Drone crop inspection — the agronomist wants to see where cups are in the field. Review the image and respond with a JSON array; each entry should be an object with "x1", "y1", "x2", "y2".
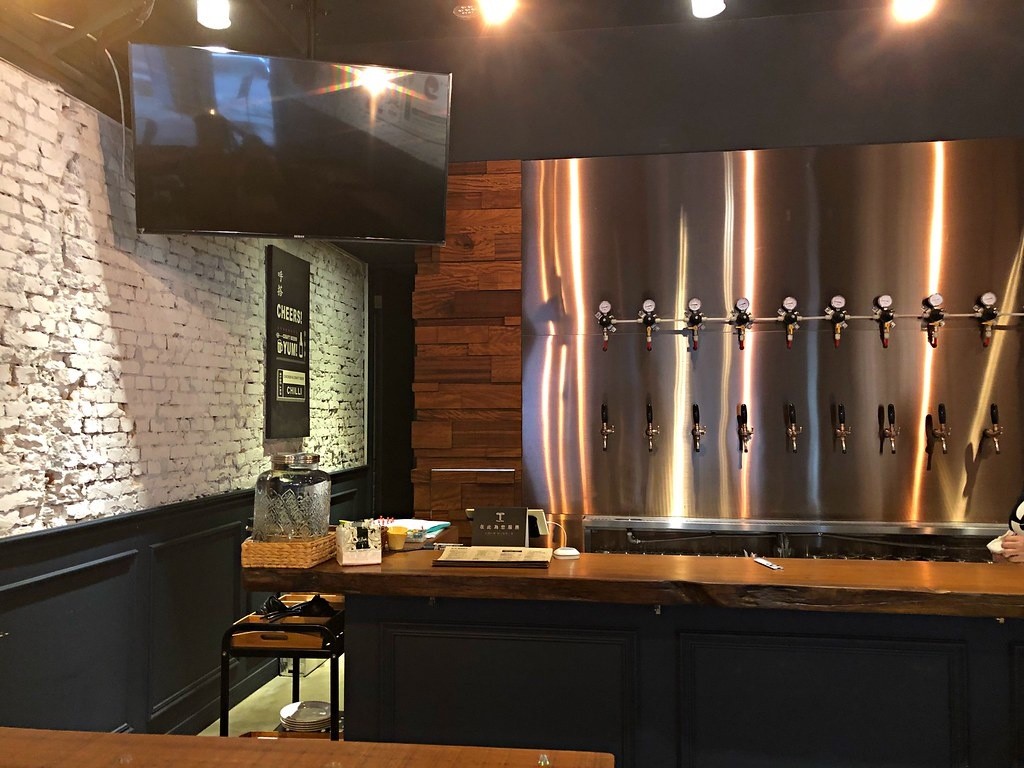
[
  {"x1": 381, "y1": 526, "x2": 389, "y2": 550},
  {"x1": 387, "y1": 526, "x2": 408, "y2": 550}
]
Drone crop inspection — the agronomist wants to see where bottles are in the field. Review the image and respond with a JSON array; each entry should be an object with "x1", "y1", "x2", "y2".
[{"x1": 253, "y1": 451, "x2": 331, "y2": 543}]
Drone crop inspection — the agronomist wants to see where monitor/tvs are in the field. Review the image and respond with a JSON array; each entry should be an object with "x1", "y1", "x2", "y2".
[{"x1": 128, "y1": 41, "x2": 452, "y2": 248}]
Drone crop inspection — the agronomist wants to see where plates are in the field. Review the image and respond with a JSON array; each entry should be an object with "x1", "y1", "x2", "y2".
[{"x1": 279, "y1": 700, "x2": 331, "y2": 732}]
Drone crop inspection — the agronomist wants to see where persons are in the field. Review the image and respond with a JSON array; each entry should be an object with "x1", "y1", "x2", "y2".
[{"x1": 991, "y1": 495, "x2": 1024, "y2": 563}]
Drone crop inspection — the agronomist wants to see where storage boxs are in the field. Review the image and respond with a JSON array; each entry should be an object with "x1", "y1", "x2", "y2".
[
  {"x1": 336, "y1": 525, "x2": 382, "y2": 566},
  {"x1": 240, "y1": 730, "x2": 345, "y2": 740},
  {"x1": 231, "y1": 593, "x2": 347, "y2": 649}
]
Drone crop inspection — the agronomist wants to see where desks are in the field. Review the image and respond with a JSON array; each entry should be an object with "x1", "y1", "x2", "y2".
[{"x1": 0, "y1": 725, "x2": 616, "y2": 768}]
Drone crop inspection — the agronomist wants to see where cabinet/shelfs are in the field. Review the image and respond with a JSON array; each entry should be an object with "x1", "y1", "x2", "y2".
[{"x1": 219, "y1": 626, "x2": 346, "y2": 741}]
